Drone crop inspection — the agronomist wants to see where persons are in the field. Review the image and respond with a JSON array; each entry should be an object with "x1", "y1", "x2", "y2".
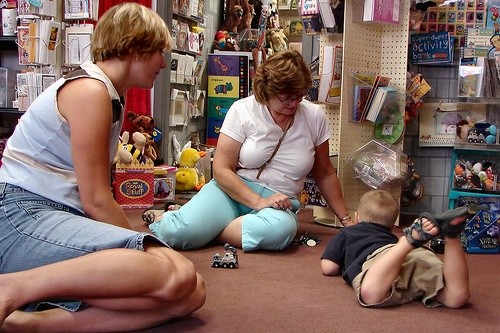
[
  {"x1": 0, "y1": 3, "x2": 207, "y2": 333},
  {"x1": 320, "y1": 190, "x2": 470, "y2": 309},
  {"x1": 140, "y1": 49, "x2": 354, "y2": 252}
]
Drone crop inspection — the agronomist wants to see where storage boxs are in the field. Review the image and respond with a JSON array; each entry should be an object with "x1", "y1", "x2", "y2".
[{"x1": 154, "y1": 164, "x2": 178, "y2": 201}]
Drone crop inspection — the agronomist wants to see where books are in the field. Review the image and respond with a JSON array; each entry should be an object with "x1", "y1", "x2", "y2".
[
  {"x1": 301, "y1": 0, "x2": 323, "y2": 35},
  {"x1": 353, "y1": 75, "x2": 398, "y2": 124}
]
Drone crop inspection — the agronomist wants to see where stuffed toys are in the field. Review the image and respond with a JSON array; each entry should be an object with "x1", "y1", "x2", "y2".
[
  {"x1": 222, "y1": 0, "x2": 278, "y2": 39},
  {"x1": 114, "y1": 111, "x2": 165, "y2": 166},
  {"x1": 173, "y1": 135, "x2": 206, "y2": 190},
  {"x1": 271, "y1": 29, "x2": 289, "y2": 51},
  {"x1": 455, "y1": 159, "x2": 500, "y2": 190}
]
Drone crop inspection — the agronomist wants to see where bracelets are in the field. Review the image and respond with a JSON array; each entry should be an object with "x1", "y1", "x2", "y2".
[{"x1": 341, "y1": 215, "x2": 351, "y2": 221}]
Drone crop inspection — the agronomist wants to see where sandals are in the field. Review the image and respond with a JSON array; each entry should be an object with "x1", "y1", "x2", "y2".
[
  {"x1": 142, "y1": 206, "x2": 169, "y2": 223},
  {"x1": 435, "y1": 207, "x2": 470, "y2": 237},
  {"x1": 164, "y1": 200, "x2": 186, "y2": 211},
  {"x1": 402, "y1": 213, "x2": 442, "y2": 249}
]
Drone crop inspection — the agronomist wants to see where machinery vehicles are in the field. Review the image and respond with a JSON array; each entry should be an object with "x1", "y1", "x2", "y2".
[{"x1": 212, "y1": 243, "x2": 239, "y2": 270}]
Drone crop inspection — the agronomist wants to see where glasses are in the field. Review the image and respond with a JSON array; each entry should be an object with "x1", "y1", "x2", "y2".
[{"x1": 273, "y1": 93, "x2": 304, "y2": 105}]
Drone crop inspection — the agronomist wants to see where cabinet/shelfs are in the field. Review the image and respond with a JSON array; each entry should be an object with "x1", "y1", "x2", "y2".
[{"x1": 301, "y1": 0, "x2": 500, "y2": 254}]
